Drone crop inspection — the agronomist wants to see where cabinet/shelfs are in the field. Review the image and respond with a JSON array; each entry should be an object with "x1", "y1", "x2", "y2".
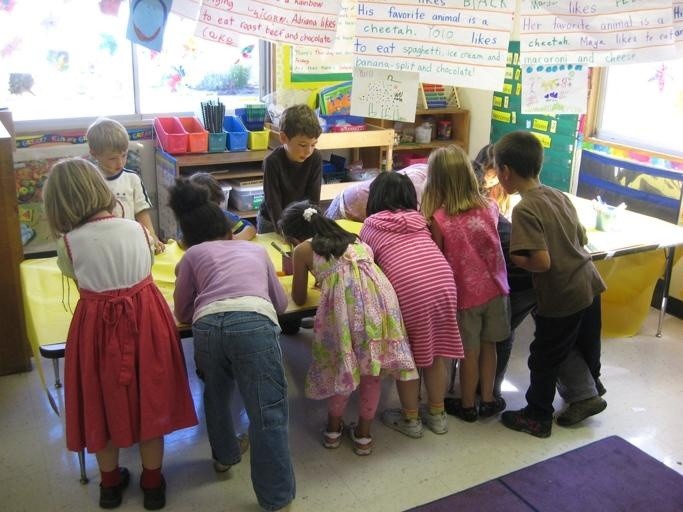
[
  {"x1": 264, "y1": 120, "x2": 395, "y2": 201},
  {"x1": 362, "y1": 108, "x2": 471, "y2": 174},
  {"x1": 154, "y1": 147, "x2": 275, "y2": 240},
  {"x1": 0, "y1": 121, "x2": 33, "y2": 377}
]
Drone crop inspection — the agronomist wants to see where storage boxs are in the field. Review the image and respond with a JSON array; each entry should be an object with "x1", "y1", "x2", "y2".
[
  {"x1": 217, "y1": 181, "x2": 232, "y2": 211},
  {"x1": 228, "y1": 180, "x2": 264, "y2": 212},
  {"x1": 401, "y1": 153, "x2": 427, "y2": 167},
  {"x1": 153, "y1": 114, "x2": 270, "y2": 157}
]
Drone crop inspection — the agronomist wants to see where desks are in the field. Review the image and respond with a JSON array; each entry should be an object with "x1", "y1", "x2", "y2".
[{"x1": 15, "y1": 215, "x2": 426, "y2": 485}]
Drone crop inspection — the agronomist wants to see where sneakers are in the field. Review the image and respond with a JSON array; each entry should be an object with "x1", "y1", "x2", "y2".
[
  {"x1": 501, "y1": 406, "x2": 554, "y2": 438},
  {"x1": 214, "y1": 432, "x2": 250, "y2": 473},
  {"x1": 479, "y1": 397, "x2": 507, "y2": 417},
  {"x1": 555, "y1": 379, "x2": 608, "y2": 428},
  {"x1": 419, "y1": 407, "x2": 449, "y2": 435},
  {"x1": 99, "y1": 466, "x2": 130, "y2": 508},
  {"x1": 349, "y1": 421, "x2": 373, "y2": 456},
  {"x1": 382, "y1": 410, "x2": 423, "y2": 438},
  {"x1": 139, "y1": 472, "x2": 166, "y2": 511},
  {"x1": 444, "y1": 397, "x2": 477, "y2": 422},
  {"x1": 321, "y1": 418, "x2": 345, "y2": 447}
]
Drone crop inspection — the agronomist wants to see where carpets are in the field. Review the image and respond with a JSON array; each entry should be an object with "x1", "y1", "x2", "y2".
[{"x1": 405, "y1": 433, "x2": 683, "y2": 511}]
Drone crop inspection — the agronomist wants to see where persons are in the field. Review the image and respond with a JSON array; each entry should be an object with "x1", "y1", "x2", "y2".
[
  {"x1": 354, "y1": 169, "x2": 467, "y2": 441},
  {"x1": 165, "y1": 174, "x2": 297, "y2": 512},
  {"x1": 465, "y1": 159, "x2": 607, "y2": 427},
  {"x1": 320, "y1": 157, "x2": 486, "y2": 223},
  {"x1": 41, "y1": 155, "x2": 201, "y2": 512},
  {"x1": 276, "y1": 198, "x2": 421, "y2": 458},
  {"x1": 255, "y1": 105, "x2": 326, "y2": 335},
  {"x1": 416, "y1": 143, "x2": 516, "y2": 424},
  {"x1": 176, "y1": 169, "x2": 259, "y2": 253},
  {"x1": 82, "y1": 117, "x2": 166, "y2": 256},
  {"x1": 469, "y1": 142, "x2": 512, "y2": 219},
  {"x1": 491, "y1": 130, "x2": 607, "y2": 439}
]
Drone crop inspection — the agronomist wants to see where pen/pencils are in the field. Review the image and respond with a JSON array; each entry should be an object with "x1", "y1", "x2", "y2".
[
  {"x1": 271, "y1": 242, "x2": 290, "y2": 258},
  {"x1": 200, "y1": 97, "x2": 225, "y2": 133},
  {"x1": 289, "y1": 243, "x2": 294, "y2": 254},
  {"x1": 246, "y1": 104, "x2": 266, "y2": 131}
]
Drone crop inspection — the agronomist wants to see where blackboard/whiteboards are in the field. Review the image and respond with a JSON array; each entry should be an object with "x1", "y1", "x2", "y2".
[{"x1": 583, "y1": 43, "x2": 683, "y2": 164}]
